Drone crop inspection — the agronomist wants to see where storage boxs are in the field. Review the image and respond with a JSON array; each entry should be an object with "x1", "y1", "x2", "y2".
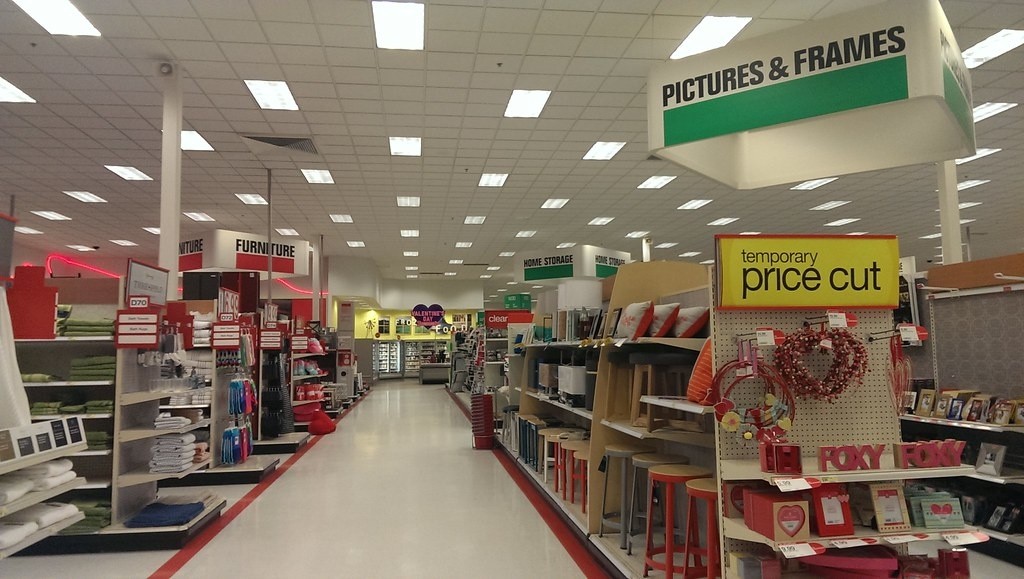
[
  {"x1": 744, "y1": 490, "x2": 811, "y2": 542},
  {"x1": 729, "y1": 552, "x2": 782, "y2": 579}
]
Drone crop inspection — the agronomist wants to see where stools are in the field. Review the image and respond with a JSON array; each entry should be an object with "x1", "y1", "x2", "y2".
[
  {"x1": 598, "y1": 444, "x2": 657, "y2": 549},
  {"x1": 519, "y1": 415, "x2": 554, "y2": 462},
  {"x1": 574, "y1": 449, "x2": 590, "y2": 514},
  {"x1": 681, "y1": 479, "x2": 722, "y2": 579},
  {"x1": 626, "y1": 453, "x2": 688, "y2": 572},
  {"x1": 538, "y1": 428, "x2": 585, "y2": 490},
  {"x1": 527, "y1": 418, "x2": 563, "y2": 473},
  {"x1": 643, "y1": 465, "x2": 713, "y2": 579},
  {"x1": 560, "y1": 440, "x2": 590, "y2": 504}
]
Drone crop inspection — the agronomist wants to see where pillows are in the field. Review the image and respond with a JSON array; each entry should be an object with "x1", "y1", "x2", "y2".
[
  {"x1": 668, "y1": 307, "x2": 710, "y2": 338},
  {"x1": 645, "y1": 303, "x2": 680, "y2": 337},
  {"x1": 615, "y1": 300, "x2": 654, "y2": 341}
]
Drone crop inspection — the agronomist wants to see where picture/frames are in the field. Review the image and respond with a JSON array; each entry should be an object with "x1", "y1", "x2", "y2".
[
  {"x1": 898, "y1": 391, "x2": 917, "y2": 411},
  {"x1": 999, "y1": 505, "x2": 1023, "y2": 535},
  {"x1": 967, "y1": 400, "x2": 984, "y2": 421},
  {"x1": 594, "y1": 312, "x2": 608, "y2": 339},
  {"x1": 984, "y1": 503, "x2": 1011, "y2": 530},
  {"x1": 811, "y1": 487, "x2": 856, "y2": 538},
  {"x1": 976, "y1": 443, "x2": 1007, "y2": 476},
  {"x1": 867, "y1": 484, "x2": 911, "y2": 533},
  {"x1": 993, "y1": 408, "x2": 1011, "y2": 423},
  {"x1": 606, "y1": 307, "x2": 622, "y2": 338},
  {"x1": 948, "y1": 398, "x2": 965, "y2": 421},
  {"x1": 933, "y1": 393, "x2": 951, "y2": 418},
  {"x1": 989, "y1": 402, "x2": 1015, "y2": 415},
  {"x1": 917, "y1": 389, "x2": 936, "y2": 417}
]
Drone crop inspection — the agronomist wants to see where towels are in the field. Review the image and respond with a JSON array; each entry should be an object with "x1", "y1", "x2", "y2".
[
  {"x1": 0, "y1": 476, "x2": 34, "y2": 503},
  {"x1": 30, "y1": 401, "x2": 65, "y2": 414},
  {"x1": 123, "y1": 502, "x2": 205, "y2": 528},
  {"x1": 193, "y1": 442, "x2": 210, "y2": 463},
  {"x1": 85, "y1": 400, "x2": 114, "y2": 414},
  {"x1": 21, "y1": 373, "x2": 64, "y2": 382},
  {"x1": 170, "y1": 409, "x2": 203, "y2": 423},
  {"x1": 56, "y1": 304, "x2": 73, "y2": 337},
  {"x1": 154, "y1": 416, "x2": 191, "y2": 429},
  {"x1": 60, "y1": 404, "x2": 84, "y2": 414},
  {"x1": 84, "y1": 431, "x2": 113, "y2": 451},
  {"x1": 0, "y1": 521, "x2": 38, "y2": 548},
  {"x1": 149, "y1": 433, "x2": 195, "y2": 473},
  {"x1": 10, "y1": 459, "x2": 75, "y2": 492},
  {"x1": 64, "y1": 316, "x2": 116, "y2": 336},
  {"x1": 60, "y1": 496, "x2": 111, "y2": 532},
  {"x1": 10, "y1": 504, "x2": 79, "y2": 528},
  {"x1": 153, "y1": 490, "x2": 216, "y2": 507},
  {"x1": 71, "y1": 355, "x2": 115, "y2": 380}
]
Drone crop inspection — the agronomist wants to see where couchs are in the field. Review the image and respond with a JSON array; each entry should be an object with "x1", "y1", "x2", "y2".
[{"x1": 418, "y1": 363, "x2": 451, "y2": 385}]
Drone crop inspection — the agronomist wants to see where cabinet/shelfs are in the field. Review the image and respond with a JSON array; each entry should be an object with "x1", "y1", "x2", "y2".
[
  {"x1": 0, "y1": 195, "x2": 400, "y2": 579},
  {"x1": 403, "y1": 238, "x2": 1024, "y2": 579}
]
